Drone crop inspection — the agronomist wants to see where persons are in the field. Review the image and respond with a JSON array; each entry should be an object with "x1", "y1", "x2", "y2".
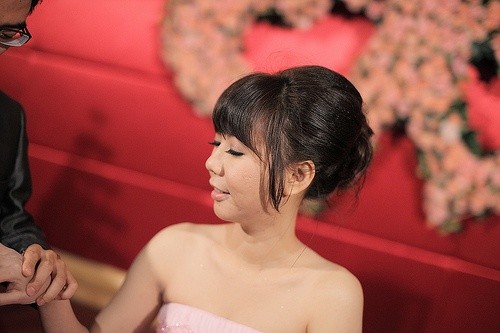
[
  {"x1": 0, "y1": 1, "x2": 78, "y2": 310},
  {"x1": 0, "y1": 64, "x2": 374, "y2": 333}
]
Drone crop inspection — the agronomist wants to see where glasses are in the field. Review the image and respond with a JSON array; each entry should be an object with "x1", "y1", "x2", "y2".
[{"x1": 0, "y1": 21, "x2": 32, "y2": 47}]
